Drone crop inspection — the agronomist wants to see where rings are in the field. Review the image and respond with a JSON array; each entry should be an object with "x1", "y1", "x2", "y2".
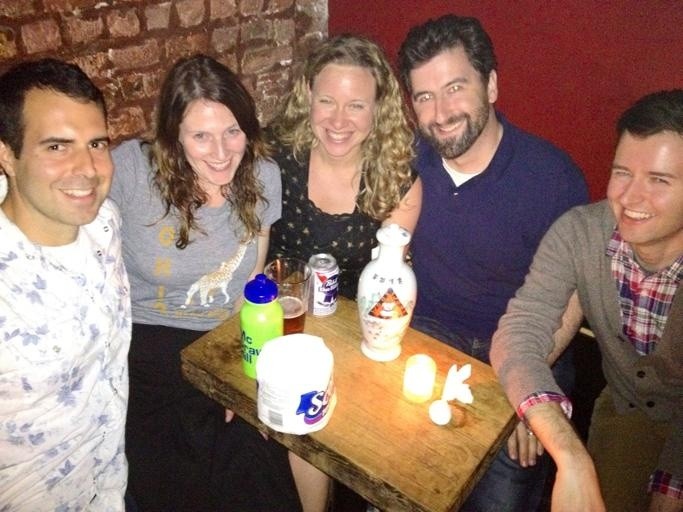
[{"x1": 528, "y1": 432, "x2": 536, "y2": 435}]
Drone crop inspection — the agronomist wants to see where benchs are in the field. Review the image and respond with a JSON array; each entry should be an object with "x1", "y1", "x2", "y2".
[{"x1": 324, "y1": 1, "x2": 683, "y2": 511}]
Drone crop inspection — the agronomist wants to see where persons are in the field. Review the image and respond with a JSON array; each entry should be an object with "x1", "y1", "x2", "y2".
[
  {"x1": 1, "y1": 56, "x2": 134, "y2": 512},
  {"x1": 396, "y1": 15, "x2": 592, "y2": 511},
  {"x1": 486, "y1": 88, "x2": 682, "y2": 511},
  {"x1": 255, "y1": 30, "x2": 416, "y2": 512},
  {"x1": 102, "y1": 50, "x2": 299, "y2": 511}
]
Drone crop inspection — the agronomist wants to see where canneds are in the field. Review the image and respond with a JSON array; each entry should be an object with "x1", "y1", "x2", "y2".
[{"x1": 304, "y1": 253, "x2": 339, "y2": 316}]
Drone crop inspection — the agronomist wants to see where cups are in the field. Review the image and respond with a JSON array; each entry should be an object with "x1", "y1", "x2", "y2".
[{"x1": 264, "y1": 258, "x2": 311, "y2": 334}]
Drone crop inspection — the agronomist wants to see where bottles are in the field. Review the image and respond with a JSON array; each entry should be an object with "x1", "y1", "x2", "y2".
[
  {"x1": 240, "y1": 272, "x2": 283, "y2": 378},
  {"x1": 357, "y1": 222, "x2": 417, "y2": 363}
]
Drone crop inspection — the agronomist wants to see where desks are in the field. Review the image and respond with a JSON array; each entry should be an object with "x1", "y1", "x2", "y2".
[{"x1": 178, "y1": 285, "x2": 523, "y2": 511}]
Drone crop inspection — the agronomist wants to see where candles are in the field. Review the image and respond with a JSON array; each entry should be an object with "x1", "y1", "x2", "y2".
[{"x1": 402, "y1": 354, "x2": 436, "y2": 402}]
